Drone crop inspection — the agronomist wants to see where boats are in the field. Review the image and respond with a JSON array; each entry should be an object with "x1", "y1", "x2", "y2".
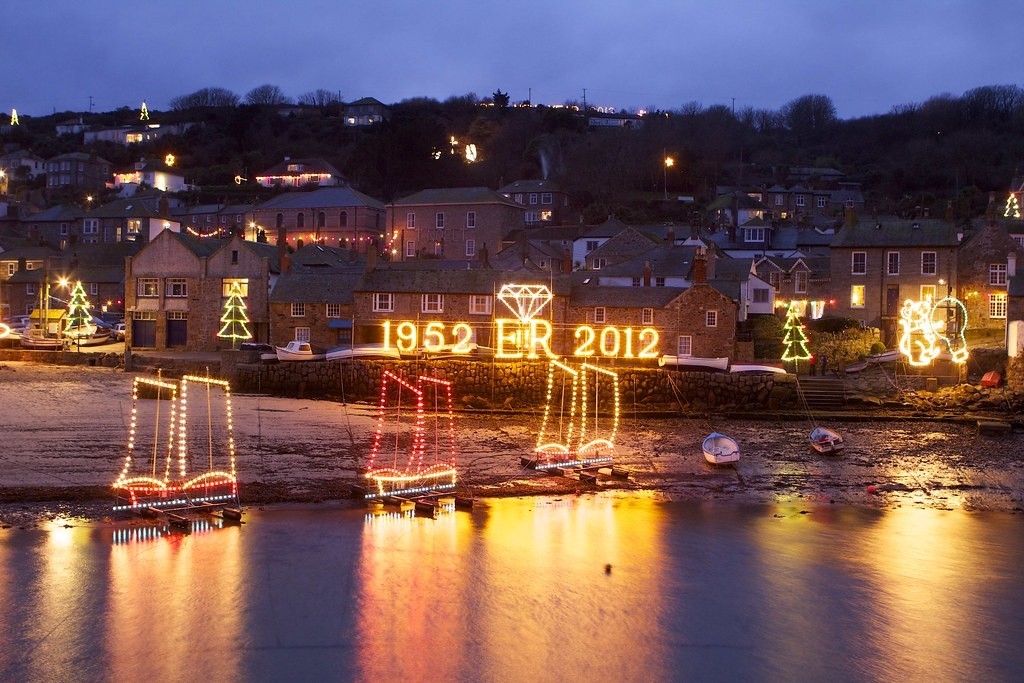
[
  {"x1": 241, "y1": 342, "x2": 277, "y2": 359},
  {"x1": 809, "y1": 426, "x2": 846, "y2": 456},
  {"x1": 701, "y1": 431, "x2": 741, "y2": 465},
  {"x1": 19, "y1": 328, "x2": 70, "y2": 349},
  {"x1": 67, "y1": 322, "x2": 97, "y2": 336},
  {"x1": 729, "y1": 364, "x2": 786, "y2": 374},
  {"x1": 74, "y1": 332, "x2": 110, "y2": 346},
  {"x1": 845, "y1": 358, "x2": 868, "y2": 373},
  {"x1": 657, "y1": 354, "x2": 729, "y2": 371},
  {"x1": 865, "y1": 349, "x2": 902, "y2": 363},
  {"x1": 275, "y1": 341, "x2": 326, "y2": 361},
  {"x1": 326, "y1": 342, "x2": 403, "y2": 359},
  {"x1": 422, "y1": 342, "x2": 481, "y2": 361}
]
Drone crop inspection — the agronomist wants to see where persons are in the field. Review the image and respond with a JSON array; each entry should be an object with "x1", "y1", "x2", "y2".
[
  {"x1": 821, "y1": 355, "x2": 828, "y2": 376},
  {"x1": 809, "y1": 353, "x2": 817, "y2": 376}
]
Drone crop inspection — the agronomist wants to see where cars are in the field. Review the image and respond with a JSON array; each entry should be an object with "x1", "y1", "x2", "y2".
[{"x1": 108, "y1": 322, "x2": 125, "y2": 340}]
